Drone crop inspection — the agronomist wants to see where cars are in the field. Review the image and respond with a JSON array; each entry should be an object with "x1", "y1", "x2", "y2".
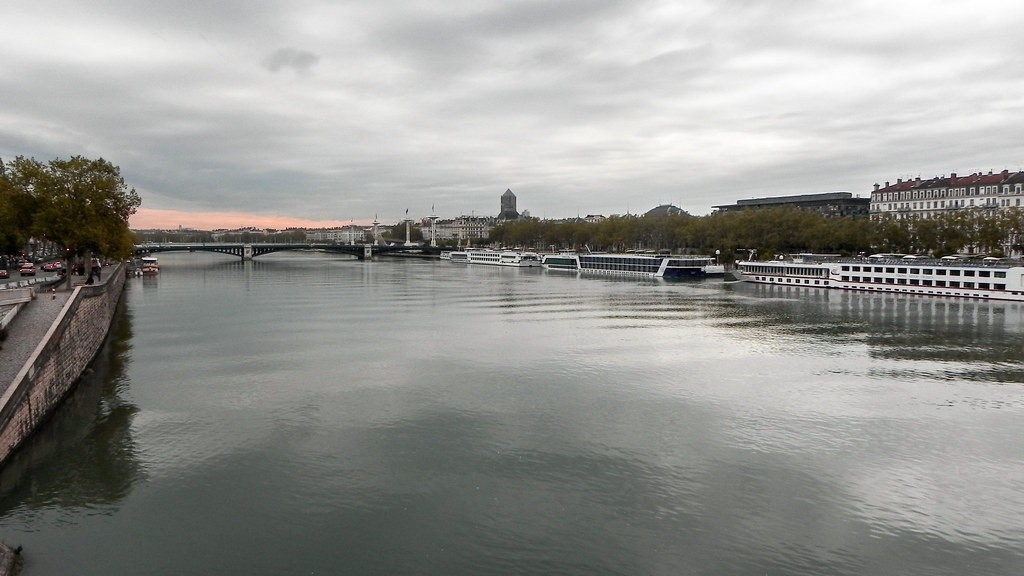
[
  {"x1": 0, "y1": 269, "x2": 10, "y2": 279},
  {"x1": 14, "y1": 247, "x2": 102, "y2": 277}
]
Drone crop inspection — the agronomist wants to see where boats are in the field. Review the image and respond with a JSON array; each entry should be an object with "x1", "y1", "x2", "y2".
[
  {"x1": 439, "y1": 248, "x2": 1024, "y2": 302},
  {"x1": 126, "y1": 255, "x2": 160, "y2": 277}
]
